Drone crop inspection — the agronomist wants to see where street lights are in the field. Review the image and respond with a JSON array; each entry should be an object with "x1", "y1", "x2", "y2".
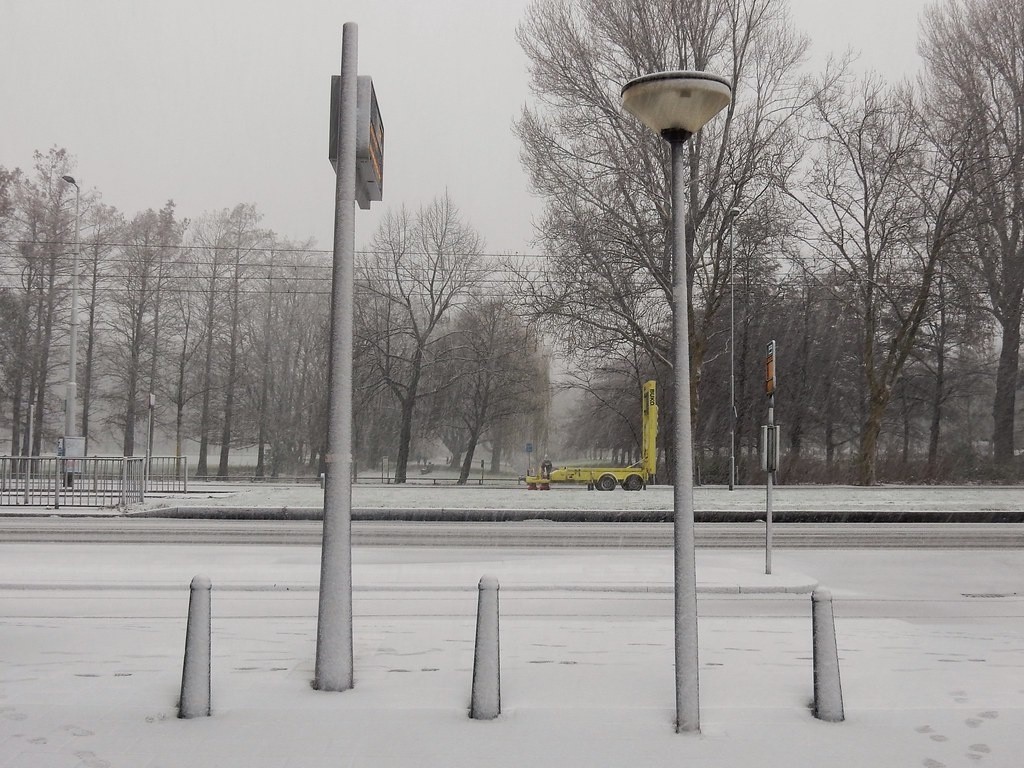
[
  {"x1": 617, "y1": 68, "x2": 738, "y2": 739},
  {"x1": 60, "y1": 172, "x2": 85, "y2": 490}
]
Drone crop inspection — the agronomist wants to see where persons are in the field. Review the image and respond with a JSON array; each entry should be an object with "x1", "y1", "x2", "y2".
[{"x1": 541, "y1": 455, "x2": 552, "y2": 484}]
